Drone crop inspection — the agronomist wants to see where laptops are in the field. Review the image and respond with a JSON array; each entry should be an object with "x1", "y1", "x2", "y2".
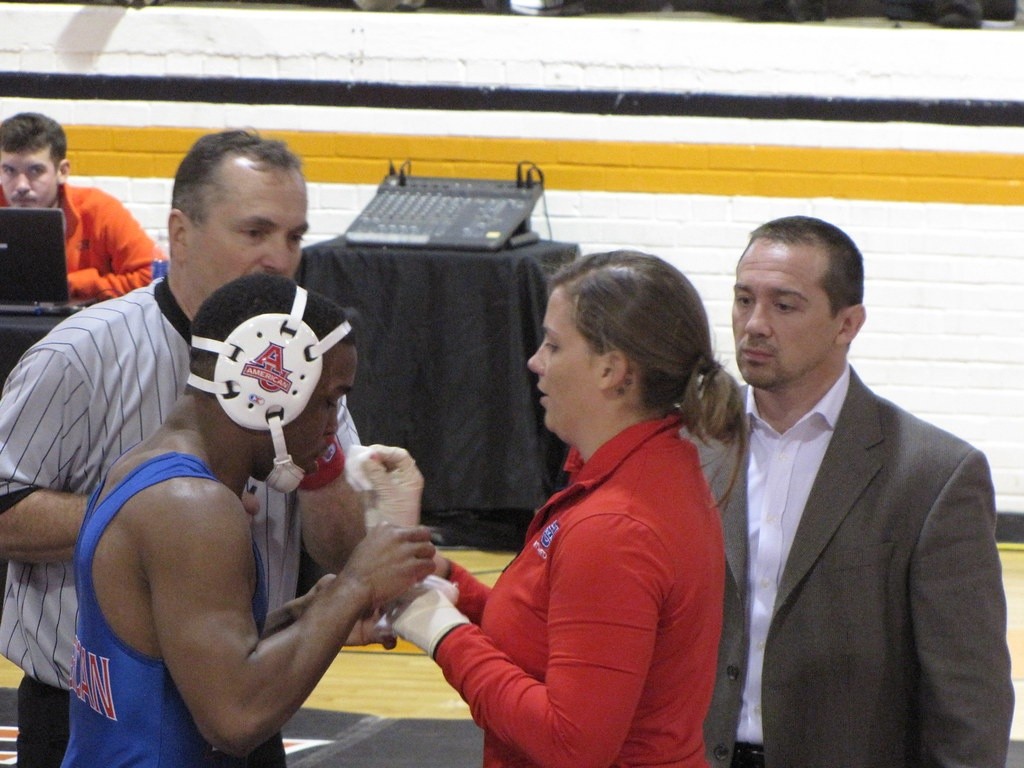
[{"x1": 0, "y1": 208, "x2": 96, "y2": 316}]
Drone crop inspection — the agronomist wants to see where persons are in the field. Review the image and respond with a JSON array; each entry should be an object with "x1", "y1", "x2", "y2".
[
  {"x1": 668, "y1": 215, "x2": 1017, "y2": 768},
  {"x1": 0, "y1": 132, "x2": 368, "y2": 768},
  {"x1": 345, "y1": 251, "x2": 743, "y2": 768},
  {"x1": 59, "y1": 276, "x2": 435, "y2": 768},
  {"x1": 0, "y1": 113, "x2": 168, "y2": 302}
]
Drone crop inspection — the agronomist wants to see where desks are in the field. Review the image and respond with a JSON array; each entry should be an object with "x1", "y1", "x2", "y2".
[{"x1": 298, "y1": 236, "x2": 577, "y2": 554}]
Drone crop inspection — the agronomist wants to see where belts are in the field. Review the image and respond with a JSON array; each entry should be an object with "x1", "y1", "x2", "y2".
[{"x1": 732, "y1": 748, "x2": 764, "y2": 768}]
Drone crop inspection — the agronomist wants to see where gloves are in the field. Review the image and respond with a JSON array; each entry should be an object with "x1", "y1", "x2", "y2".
[{"x1": 374, "y1": 576, "x2": 473, "y2": 658}]
